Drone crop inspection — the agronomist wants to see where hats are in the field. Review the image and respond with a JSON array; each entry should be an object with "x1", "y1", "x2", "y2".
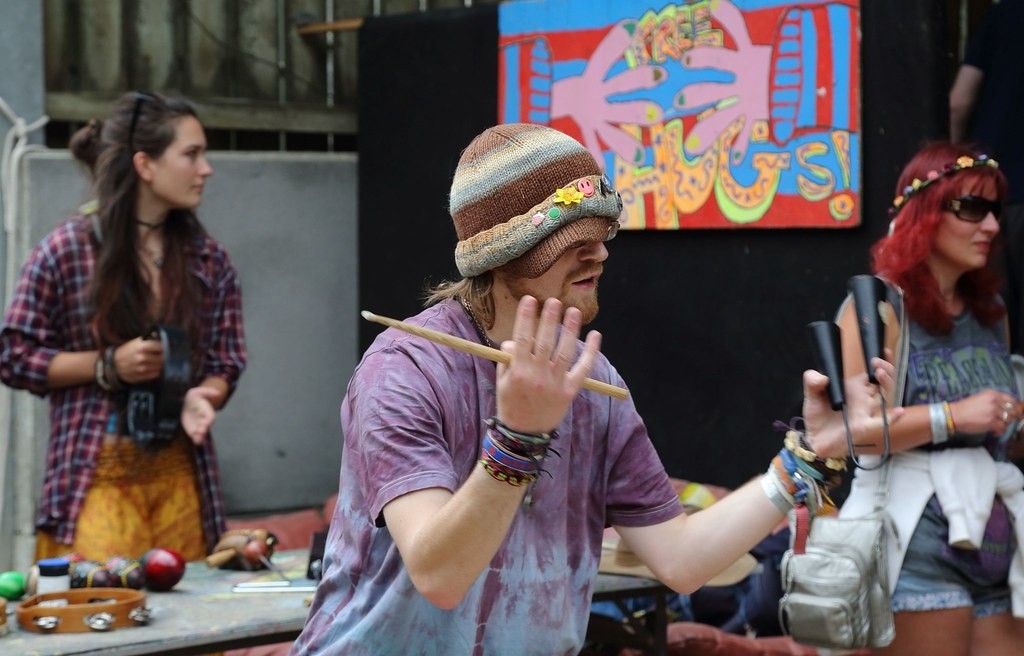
[{"x1": 449, "y1": 122, "x2": 623, "y2": 277}]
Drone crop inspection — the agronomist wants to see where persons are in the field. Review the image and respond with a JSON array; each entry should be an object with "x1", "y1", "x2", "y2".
[
  {"x1": 948, "y1": 1, "x2": 1024, "y2": 356},
  {"x1": 291, "y1": 123, "x2": 905, "y2": 656},
  {"x1": 837, "y1": 144, "x2": 1024, "y2": 656},
  {"x1": 0, "y1": 92, "x2": 269, "y2": 589}
]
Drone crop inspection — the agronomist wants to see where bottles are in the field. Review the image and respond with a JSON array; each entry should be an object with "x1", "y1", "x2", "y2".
[{"x1": 36, "y1": 558, "x2": 72, "y2": 622}]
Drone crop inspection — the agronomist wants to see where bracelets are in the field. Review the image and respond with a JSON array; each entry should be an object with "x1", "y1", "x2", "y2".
[
  {"x1": 94, "y1": 347, "x2": 118, "y2": 389},
  {"x1": 480, "y1": 417, "x2": 562, "y2": 487},
  {"x1": 929, "y1": 400, "x2": 956, "y2": 444},
  {"x1": 763, "y1": 418, "x2": 848, "y2": 555}
]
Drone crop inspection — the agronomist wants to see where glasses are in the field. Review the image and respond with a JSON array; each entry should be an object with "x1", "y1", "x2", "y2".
[
  {"x1": 570, "y1": 222, "x2": 620, "y2": 248},
  {"x1": 940, "y1": 193, "x2": 1003, "y2": 222}
]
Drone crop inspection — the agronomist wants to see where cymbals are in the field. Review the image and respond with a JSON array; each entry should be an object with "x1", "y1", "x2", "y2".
[{"x1": 16, "y1": 589, "x2": 150, "y2": 634}]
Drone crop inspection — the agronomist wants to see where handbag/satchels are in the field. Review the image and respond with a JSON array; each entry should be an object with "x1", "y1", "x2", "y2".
[{"x1": 780, "y1": 515, "x2": 894, "y2": 649}]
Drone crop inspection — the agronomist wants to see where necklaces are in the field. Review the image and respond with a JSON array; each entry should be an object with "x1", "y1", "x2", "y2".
[
  {"x1": 137, "y1": 218, "x2": 163, "y2": 229},
  {"x1": 466, "y1": 299, "x2": 541, "y2": 505}
]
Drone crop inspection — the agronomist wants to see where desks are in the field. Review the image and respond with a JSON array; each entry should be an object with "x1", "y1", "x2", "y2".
[{"x1": 1, "y1": 547, "x2": 679, "y2": 656}]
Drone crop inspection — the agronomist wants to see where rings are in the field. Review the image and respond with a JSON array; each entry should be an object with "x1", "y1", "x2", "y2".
[
  {"x1": 1002, "y1": 411, "x2": 1007, "y2": 423},
  {"x1": 1006, "y1": 403, "x2": 1011, "y2": 412}
]
